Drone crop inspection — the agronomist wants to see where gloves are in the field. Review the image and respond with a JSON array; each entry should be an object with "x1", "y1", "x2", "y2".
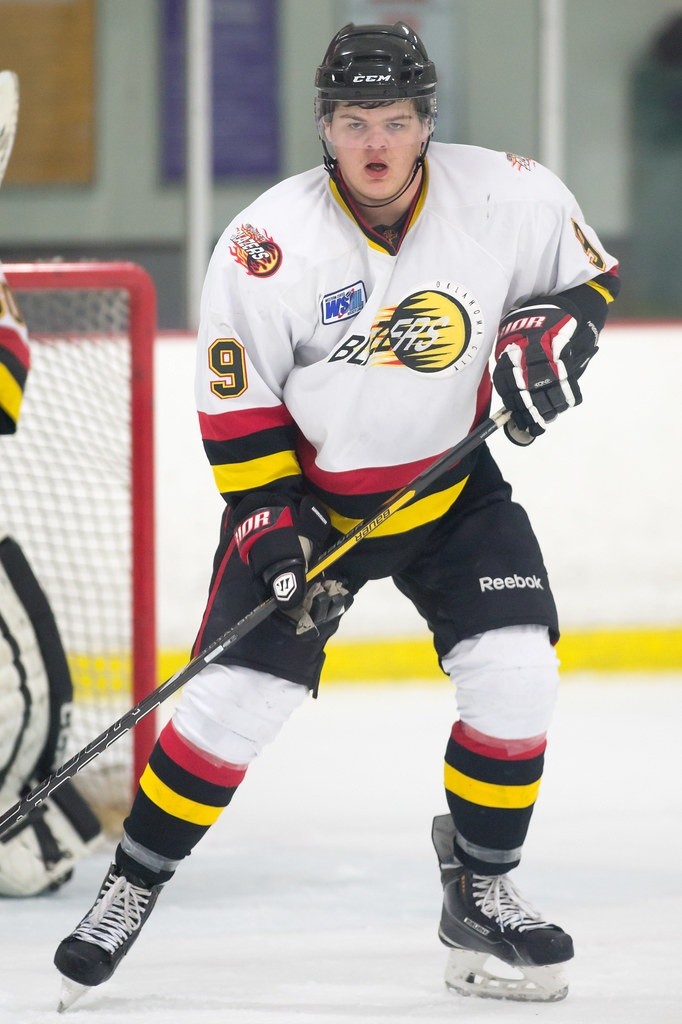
[
  {"x1": 495, "y1": 296, "x2": 600, "y2": 445},
  {"x1": 232, "y1": 493, "x2": 335, "y2": 621}
]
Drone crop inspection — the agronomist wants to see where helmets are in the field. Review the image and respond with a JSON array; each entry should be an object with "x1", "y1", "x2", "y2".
[{"x1": 315, "y1": 21, "x2": 435, "y2": 118}]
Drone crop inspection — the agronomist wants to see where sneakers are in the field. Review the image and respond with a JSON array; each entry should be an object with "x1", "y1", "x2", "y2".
[
  {"x1": 437, "y1": 860, "x2": 573, "y2": 1003},
  {"x1": 51, "y1": 863, "x2": 165, "y2": 1014}
]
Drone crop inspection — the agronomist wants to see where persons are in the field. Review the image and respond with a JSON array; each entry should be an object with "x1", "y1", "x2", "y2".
[
  {"x1": 45, "y1": 21, "x2": 619, "y2": 1013},
  {"x1": 0, "y1": 69, "x2": 105, "y2": 897}
]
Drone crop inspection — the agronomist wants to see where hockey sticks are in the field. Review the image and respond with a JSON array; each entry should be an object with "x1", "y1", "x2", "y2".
[{"x1": 0, "y1": 400, "x2": 515, "y2": 847}]
